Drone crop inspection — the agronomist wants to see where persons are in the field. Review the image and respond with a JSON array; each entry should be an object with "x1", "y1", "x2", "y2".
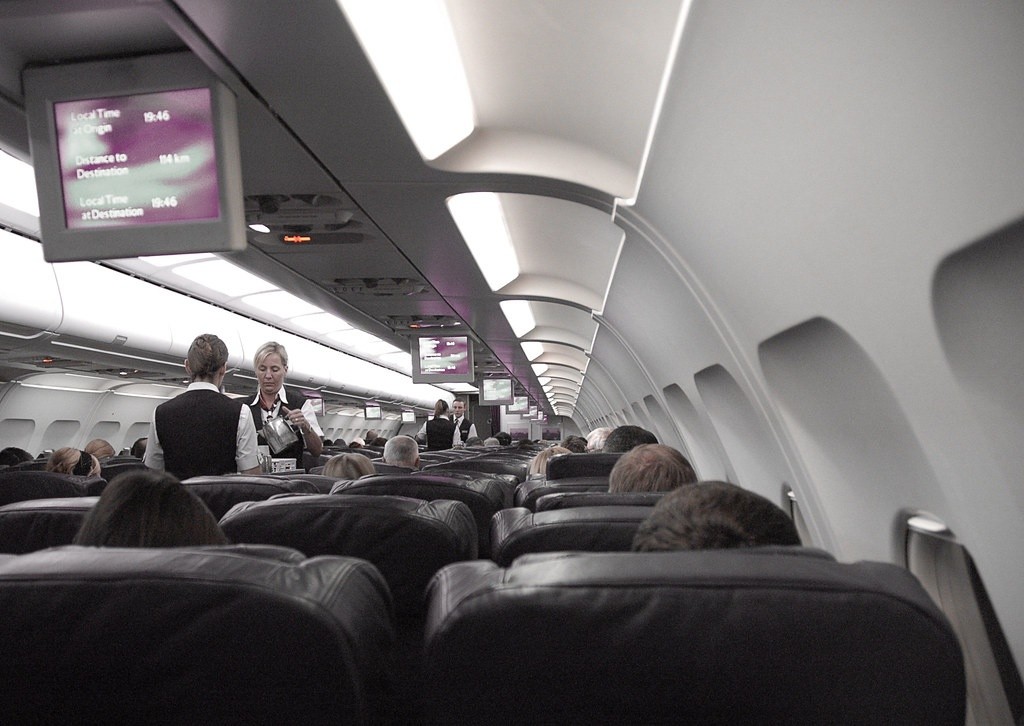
[
  {"x1": 0, "y1": 333, "x2": 662, "y2": 484},
  {"x1": 630, "y1": 481, "x2": 798, "y2": 553},
  {"x1": 607, "y1": 442, "x2": 702, "y2": 499}
]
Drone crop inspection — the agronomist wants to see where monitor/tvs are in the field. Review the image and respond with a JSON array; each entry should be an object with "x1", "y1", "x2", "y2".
[
  {"x1": 427, "y1": 415, "x2": 435, "y2": 421},
  {"x1": 506, "y1": 395, "x2": 530, "y2": 414},
  {"x1": 528, "y1": 410, "x2": 548, "y2": 424},
  {"x1": 410, "y1": 335, "x2": 474, "y2": 384},
  {"x1": 520, "y1": 405, "x2": 538, "y2": 419},
  {"x1": 478, "y1": 377, "x2": 514, "y2": 405},
  {"x1": 401, "y1": 411, "x2": 416, "y2": 424},
  {"x1": 364, "y1": 406, "x2": 382, "y2": 420},
  {"x1": 306, "y1": 397, "x2": 323, "y2": 416},
  {"x1": 21, "y1": 50, "x2": 247, "y2": 263}
]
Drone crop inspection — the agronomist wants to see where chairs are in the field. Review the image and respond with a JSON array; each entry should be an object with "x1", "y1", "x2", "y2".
[{"x1": 0, "y1": 401, "x2": 965, "y2": 726}]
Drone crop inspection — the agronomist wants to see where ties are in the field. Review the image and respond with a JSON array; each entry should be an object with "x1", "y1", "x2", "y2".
[{"x1": 454, "y1": 418, "x2": 459, "y2": 424}]
